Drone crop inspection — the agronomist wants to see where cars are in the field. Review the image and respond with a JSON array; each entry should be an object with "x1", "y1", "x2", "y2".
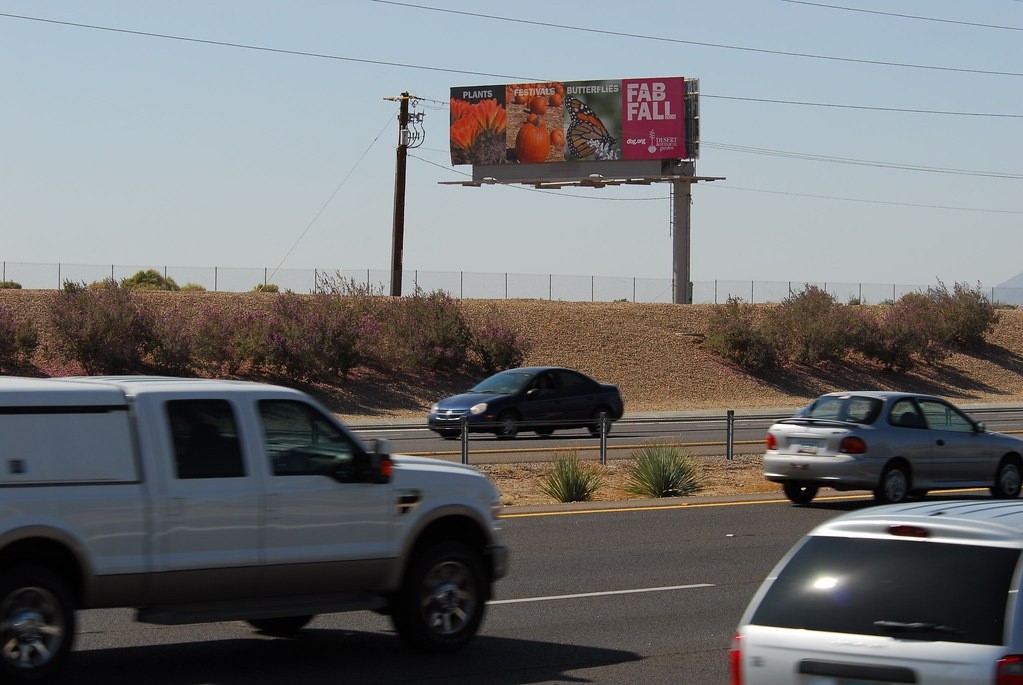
[
  {"x1": 762, "y1": 390, "x2": 1023, "y2": 506},
  {"x1": 427, "y1": 366, "x2": 624, "y2": 441},
  {"x1": 729, "y1": 499, "x2": 1023, "y2": 685}
]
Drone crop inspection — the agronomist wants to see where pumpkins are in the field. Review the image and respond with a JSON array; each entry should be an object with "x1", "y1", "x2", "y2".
[{"x1": 506, "y1": 82, "x2": 565, "y2": 163}]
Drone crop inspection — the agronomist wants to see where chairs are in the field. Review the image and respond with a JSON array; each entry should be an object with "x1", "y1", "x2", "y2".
[{"x1": 899, "y1": 411, "x2": 921, "y2": 427}]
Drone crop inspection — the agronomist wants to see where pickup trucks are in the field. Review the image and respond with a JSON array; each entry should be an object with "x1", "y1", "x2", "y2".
[{"x1": 0, "y1": 372, "x2": 511, "y2": 683}]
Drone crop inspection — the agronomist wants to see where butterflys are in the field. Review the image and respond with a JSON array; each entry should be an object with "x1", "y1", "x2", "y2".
[{"x1": 564, "y1": 92, "x2": 621, "y2": 161}]
